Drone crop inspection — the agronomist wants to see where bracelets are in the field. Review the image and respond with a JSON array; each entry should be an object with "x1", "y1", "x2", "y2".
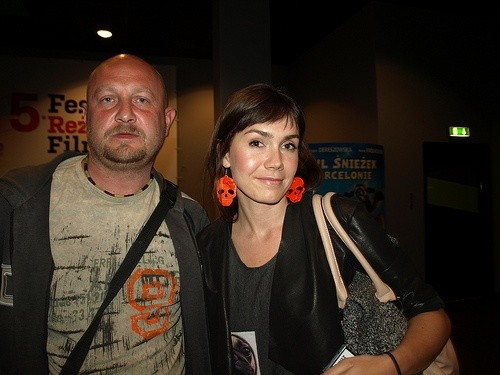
[{"x1": 384, "y1": 351, "x2": 402, "y2": 375}]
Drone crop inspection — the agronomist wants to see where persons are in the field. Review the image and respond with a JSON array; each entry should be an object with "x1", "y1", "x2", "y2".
[
  {"x1": 196, "y1": 82, "x2": 450, "y2": 375},
  {"x1": 0, "y1": 51, "x2": 213, "y2": 374}
]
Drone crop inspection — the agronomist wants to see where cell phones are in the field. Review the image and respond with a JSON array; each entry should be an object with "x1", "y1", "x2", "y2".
[{"x1": 322, "y1": 344, "x2": 356, "y2": 373}]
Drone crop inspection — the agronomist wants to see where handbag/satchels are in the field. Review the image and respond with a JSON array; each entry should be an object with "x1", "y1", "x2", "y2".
[{"x1": 313, "y1": 193, "x2": 459, "y2": 375}]
